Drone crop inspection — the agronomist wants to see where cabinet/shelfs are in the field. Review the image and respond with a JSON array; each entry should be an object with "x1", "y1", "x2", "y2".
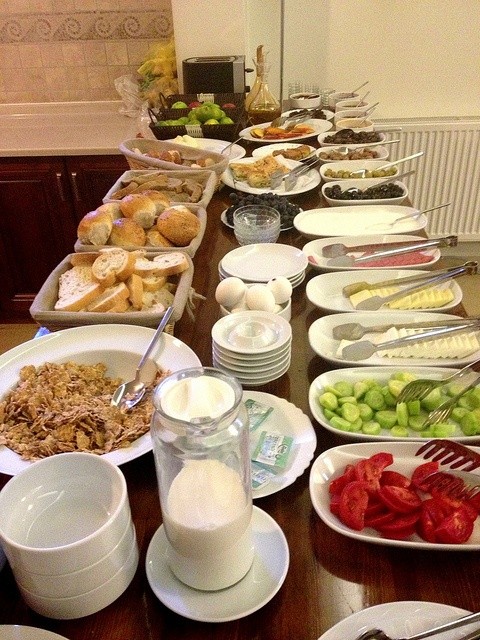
[{"x1": 1, "y1": 152, "x2": 128, "y2": 324}]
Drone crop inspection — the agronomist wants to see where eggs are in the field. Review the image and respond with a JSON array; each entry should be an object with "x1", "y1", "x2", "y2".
[
  {"x1": 271, "y1": 304, "x2": 284, "y2": 315},
  {"x1": 215, "y1": 276, "x2": 244, "y2": 307},
  {"x1": 245, "y1": 283, "x2": 274, "y2": 310},
  {"x1": 230, "y1": 303, "x2": 250, "y2": 314},
  {"x1": 267, "y1": 276, "x2": 293, "y2": 304}
]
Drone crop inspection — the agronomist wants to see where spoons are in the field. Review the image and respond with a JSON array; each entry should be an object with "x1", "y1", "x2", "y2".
[
  {"x1": 337, "y1": 128, "x2": 400, "y2": 137},
  {"x1": 349, "y1": 152, "x2": 425, "y2": 174},
  {"x1": 110, "y1": 305, "x2": 174, "y2": 419},
  {"x1": 332, "y1": 140, "x2": 401, "y2": 155},
  {"x1": 348, "y1": 169, "x2": 416, "y2": 193},
  {"x1": 358, "y1": 612, "x2": 480, "y2": 640}
]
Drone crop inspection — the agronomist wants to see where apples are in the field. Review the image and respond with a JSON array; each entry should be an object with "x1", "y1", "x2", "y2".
[{"x1": 155, "y1": 99, "x2": 236, "y2": 127}]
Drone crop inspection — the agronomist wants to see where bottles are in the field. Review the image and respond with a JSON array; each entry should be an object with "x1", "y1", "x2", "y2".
[
  {"x1": 244, "y1": 45, "x2": 275, "y2": 114},
  {"x1": 247, "y1": 52, "x2": 281, "y2": 122},
  {"x1": 148, "y1": 366, "x2": 258, "y2": 592}
]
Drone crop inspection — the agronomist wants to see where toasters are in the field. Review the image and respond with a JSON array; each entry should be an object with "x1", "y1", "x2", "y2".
[{"x1": 181, "y1": 55, "x2": 254, "y2": 95}]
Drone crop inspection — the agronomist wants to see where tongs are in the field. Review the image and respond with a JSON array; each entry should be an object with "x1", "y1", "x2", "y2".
[
  {"x1": 344, "y1": 260, "x2": 480, "y2": 309},
  {"x1": 325, "y1": 235, "x2": 459, "y2": 268},
  {"x1": 111, "y1": 172, "x2": 207, "y2": 203},
  {"x1": 271, "y1": 154, "x2": 321, "y2": 192},
  {"x1": 271, "y1": 109, "x2": 327, "y2": 132},
  {"x1": 394, "y1": 359, "x2": 480, "y2": 427},
  {"x1": 416, "y1": 438, "x2": 480, "y2": 502},
  {"x1": 333, "y1": 316, "x2": 479, "y2": 361}
]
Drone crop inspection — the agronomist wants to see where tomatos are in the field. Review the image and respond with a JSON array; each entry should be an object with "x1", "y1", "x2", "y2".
[{"x1": 327, "y1": 452, "x2": 480, "y2": 544}]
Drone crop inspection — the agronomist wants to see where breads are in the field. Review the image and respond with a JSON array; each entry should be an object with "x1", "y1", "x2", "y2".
[
  {"x1": 95, "y1": 202, "x2": 122, "y2": 219},
  {"x1": 108, "y1": 218, "x2": 147, "y2": 247},
  {"x1": 157, "y1": 205, "x2": 200, "y2": 246},
  {"x1": 145, "y1": 224, "x2": 175, "y2": 247},
  {"x1": 76, "y1": 210, "x2": 113, "y2": 247},
  {"x1": 54, "y1": 247, "x2": 190, "y2": 312},
  {"x1": 121, "y1": 194, "x2": 155, "y2": 228},
  {"x1": 144, "y1": 190, "x2": 171, "y2": 215}
]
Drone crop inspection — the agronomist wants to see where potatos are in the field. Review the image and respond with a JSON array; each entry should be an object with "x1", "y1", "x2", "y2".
[{"x1": 249, "y1": 123, "x2": 315, "y2": 139}]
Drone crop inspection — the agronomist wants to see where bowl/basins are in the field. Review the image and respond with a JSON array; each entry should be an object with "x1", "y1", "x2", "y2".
[
  {"x1": 0, "y1": 449, "x2": 139, "y2": 621},
  {"x1": 233, "y1": 205, "x2": 281, "y2": 245},
  {"x1": 1, "y1": 325, "x2": 207, "y2": 479},
  {"x1": 336, "y1": 100, "x2": 369, "y2": 111},
  {"x1": 289, "y1": 92, "x2": 322, "y2": 107},
  {"x1": 334, "y1": 109, "x2": 373, "y2": 124},
  {"x1": 119, "y1": 138, "x2": 228, "y2": 183},
  {"x1": 336, "y1": 119, "x2": 374, "y2": 133},
  {"x1": 328, "y1": 90, "x2": 361, "y2": 109},
  {"x1": 164, "y1": 137, "x2": 247, "y2": 162},
  {"x1": 215, "y1": 283, "x2": 292, "y2": 321}
]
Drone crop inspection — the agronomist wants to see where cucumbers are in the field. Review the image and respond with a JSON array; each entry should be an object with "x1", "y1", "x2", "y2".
[{"x1": 318, "y1": 373, "x2": 480, "y2": 436}]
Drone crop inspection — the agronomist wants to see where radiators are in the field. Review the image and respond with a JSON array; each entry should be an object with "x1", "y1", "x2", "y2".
[{"x1": 371, "y1": 116, "x2": 478, "y2": 242}]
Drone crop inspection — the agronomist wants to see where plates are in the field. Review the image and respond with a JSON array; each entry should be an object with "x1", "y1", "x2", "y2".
[
  {"x1": 308, "y1": 311, "x2": 480, "y2": 364},
  {"x1": 306, "y1": 367, "x2": 480, "y2": 444},
  {"x1": 239, "y1": 118, "x2": 334, "y2": 143},
  {"x1": 316, "y1": 599, "x2": 480, "y2": 640},
  {"x1": 202, "y1": 390, "x2": 316, "y2": 500},
  {"x1": 281, "y1": 107, "x2": 334, "y2": 122},
  {"x1": 222, "y1": 156, "x2": 321, "y2": 197},
  {"x1": 209, "y1": 311, "x2": 293, "y2": 391},
  {"x1": 304, "y1": 235, "x2": 441, "y2": 273},
  {"x1": 30, "y1": 252, "x2": 195, "y2": 327},
  {"x1": 220, "y1": 198, "x2": 303, "y2": 234},
  {"x1": 219, "y1": 243, "x2": 308, "y2": 290},
  {"x1": 316, "y1": 130, "x2": 385, "y2": 146},
  {"x1": 322, "y1": 180, "x2": 407, "y2": 205},
  {"x1": 293, "y1": 205, "x2": 428, "y2": 235},
  {"x1": 316, "y1": 146, "x2": 388, "y2": 162},
  {"x1": 103, "y1": 170, "x2": 218, "y2": 205},
  {"x1": 143, "y1": 502, "x2": 290, "y2": 624},
  {"x1": 306, "y1": 271, "x2": 463, "y2": 313},
  {"x1": 251, "y1": 143, "x2": 316, "y2": 160},
  {"x1": 74, "y1": 203, "x2": 207, "y2": 257},
  {"x1": 310, "y1": 442, "x2": 480, "y2": 551},
  {"x1": 320, "y1": 160, "x2": 399, "y2": 179}
]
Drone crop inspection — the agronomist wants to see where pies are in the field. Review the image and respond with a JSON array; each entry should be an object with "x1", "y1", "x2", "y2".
[{"x1": 228, "y1": 156, "x2": 291, "y2": 189}]
